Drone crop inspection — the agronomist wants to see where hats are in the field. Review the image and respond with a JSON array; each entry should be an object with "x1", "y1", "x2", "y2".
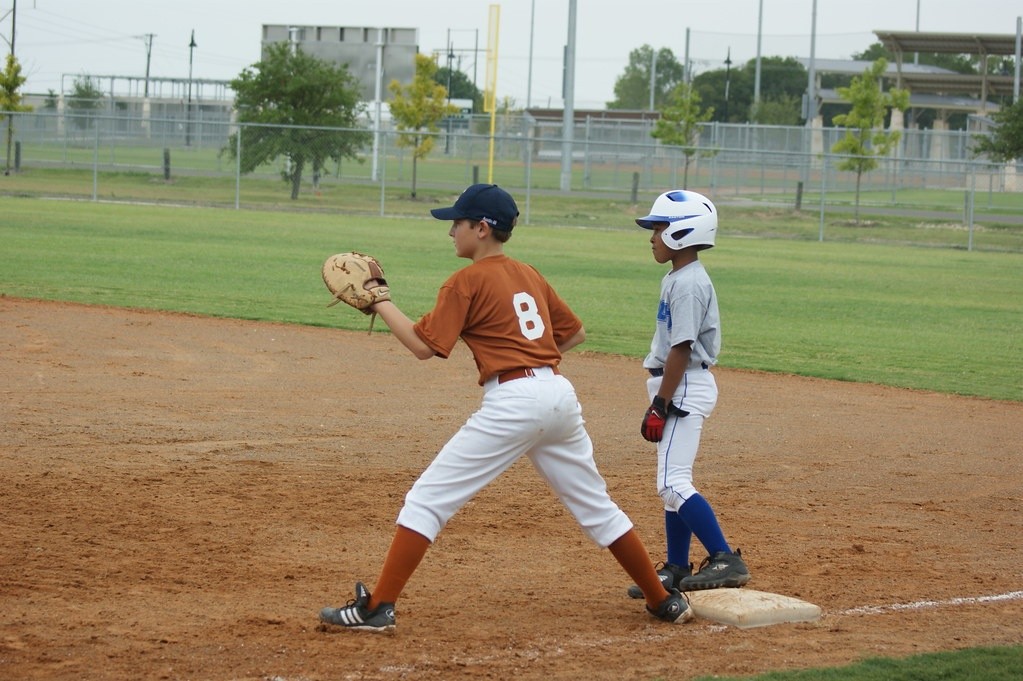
[{"x1": 430, "y1": 183, "x2": 520, "y2": 232}]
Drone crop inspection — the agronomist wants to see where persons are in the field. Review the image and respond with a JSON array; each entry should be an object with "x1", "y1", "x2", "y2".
[
  {"x1": 628, "y1": 188, "x2": 751, "y2": 598},
  {"x1": 318, "y1": 182, "x2": 697, "y2": 634}
]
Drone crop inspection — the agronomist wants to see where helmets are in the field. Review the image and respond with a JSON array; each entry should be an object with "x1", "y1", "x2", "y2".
[{"x1": 635, "y1": 189, "x2": 718, "y2": 250}]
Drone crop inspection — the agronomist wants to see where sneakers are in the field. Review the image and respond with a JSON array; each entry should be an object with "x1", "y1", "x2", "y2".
[
  {"x1": 628, "y1": 549, "x2": 753, "y2": 599},
  {"x1": 320, "y1": 581, "x2": 396, "y2": 632},
  {"x1": 645, "y1": 588, "x2": 693, "y2": 625}
]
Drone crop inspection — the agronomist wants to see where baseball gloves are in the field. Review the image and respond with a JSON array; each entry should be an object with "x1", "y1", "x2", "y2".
[{"x1": 320, "y1": 243, "x2": 392, "y2": 339}]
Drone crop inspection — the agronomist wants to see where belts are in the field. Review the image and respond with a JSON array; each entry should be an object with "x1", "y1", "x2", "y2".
[
  {"x1": 498, "y1": 366, "x2": 564, "y2": 385},
  {"x1": 649, "y1": 361, "x2": 709, "y2": 377}
]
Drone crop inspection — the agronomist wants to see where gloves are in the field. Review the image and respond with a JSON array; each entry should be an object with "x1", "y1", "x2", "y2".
[{"x1": 640, "y1": 395, "x2": 689, "y2": 443}]
